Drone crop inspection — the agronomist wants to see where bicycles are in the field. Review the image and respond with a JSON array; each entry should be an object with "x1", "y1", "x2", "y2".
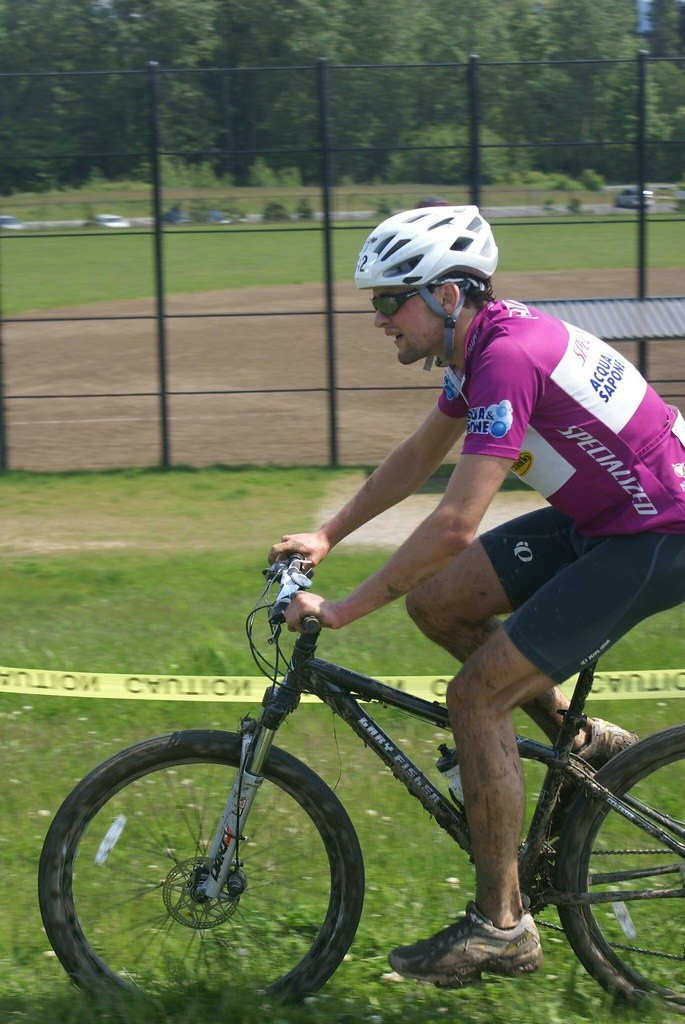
[{"x1": 36, "y1": 555, "x2": 685, "y2": 1016}]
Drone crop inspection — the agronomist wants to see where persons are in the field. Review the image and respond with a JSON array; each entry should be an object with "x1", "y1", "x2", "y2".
[{"x1": 267, "y1": 204, "x2": 685, "y2": 981}]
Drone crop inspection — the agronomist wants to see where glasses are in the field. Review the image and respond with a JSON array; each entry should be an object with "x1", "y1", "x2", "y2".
[{"x1": 371, "y1": 287, "x2": 440, "y2": 316}]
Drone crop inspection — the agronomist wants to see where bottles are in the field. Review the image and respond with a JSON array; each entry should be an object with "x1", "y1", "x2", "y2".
[{"x1": 436, "y1": 744, "x2": 463, "y2": 805}]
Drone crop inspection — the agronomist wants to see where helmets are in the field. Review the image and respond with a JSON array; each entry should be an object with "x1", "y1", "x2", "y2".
[{"x1": 354, "y1": 204, "x2": 498, "y2": 290}]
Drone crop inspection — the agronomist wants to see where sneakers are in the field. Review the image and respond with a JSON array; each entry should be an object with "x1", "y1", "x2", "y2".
[
  {"x1": 554, "y1": 717, "x2": 640, "y2": 809},
  {"x1": 387, "y1": 893, "x2": 544, "y2": 983}
]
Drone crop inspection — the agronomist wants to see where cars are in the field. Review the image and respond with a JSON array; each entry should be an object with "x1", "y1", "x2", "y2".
[
  {"x1": 616, "y1": 187, "x2": 656, "y2": 209},
  {"x1": 418, "y1": 196, "x2": 448, "y2": 211},
  {"x1": 162, "y1": 208, "x2": 194, "y2": 224},
  {"x1": 206, "y1": 213, "x2": 234, "y2": 225},
  {"x1": 1, "y1": 216, "x2": 27, "y2": 234},
  {"x1": 93, "y1": 212, "x2": 131, "y2": 228}
]
